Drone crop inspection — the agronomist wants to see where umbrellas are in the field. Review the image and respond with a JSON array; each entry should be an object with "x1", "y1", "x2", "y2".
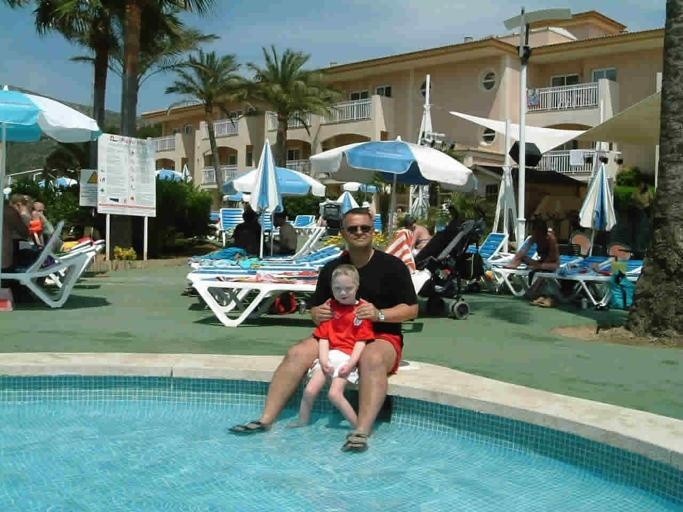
[
  {"x1": 309, "y1": 136, "x2": 473, "y2": 233},
  {"x1": 0, "y1": 83, "x2": 103, "y2": 236},
  {"x1": 341, "y1": 181, "x2": 391, "y2": 202},
  {"x1": 335, "y1": 188, "x2": 360, "y2": 216},
  {"x1": 220, "y1": 165, "x2": 327, "y2": 251},
  {"x1": 575, "y1": 161, "x2": 618, "y2": 260},
  {"x1": 155, "y1": 166, "x2": 193, "y2": 185},
  {"x1": 248, "y1": 136, "x2": 285, "y2": 260}
]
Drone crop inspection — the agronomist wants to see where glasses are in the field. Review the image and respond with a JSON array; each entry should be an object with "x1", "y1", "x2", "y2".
[{"x1": 343, "y1": 225, "x2": 374, "y2": 234}]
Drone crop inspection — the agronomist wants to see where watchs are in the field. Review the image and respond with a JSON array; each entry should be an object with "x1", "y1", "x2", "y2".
[{"x1": 377, "y1": 309, "x2": 385, "y2": 322}]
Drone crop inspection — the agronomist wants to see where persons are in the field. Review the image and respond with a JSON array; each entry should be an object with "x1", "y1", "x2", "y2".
[
  {"x1": 231, "y1": 208, "x2": 268, "y2": 253},
  {"x1": 286, "y1": 264, "x2": 375, "y2": 429},
  {"x1": 502, "y1": 220, "x2": 559, "y2": 271},
  {"x1": 0, "y1": 193, "x2": 94, "y2": 304},
  {"x1": 402, "y1": 215, "x2": 431, "y2": 258},
  {"x1": 396, "y1": 205, "x2": 405, "y2": 215},
  {"x1": 269, "y1": 213, "x2": 297, "y2": 255},
  {"x1": 227, "y1": 208, "x2": 419, "y2": 452}
]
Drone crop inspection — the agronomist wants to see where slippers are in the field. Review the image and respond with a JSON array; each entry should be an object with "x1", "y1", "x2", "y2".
[
  {"x1": 343, "y1": 432, "x2": 369, "y2": 451},
  {"x1": 529, "y1": 296, "x2": 545, "y2": 306},
  {"x1": 228, "y1": 420, "x2": 270, "y2": 435},
  {"x1": 538, "y1": 297, "x2": 560, "y2": 308}
]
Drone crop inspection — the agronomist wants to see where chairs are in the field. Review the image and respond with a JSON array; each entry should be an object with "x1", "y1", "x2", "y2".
[{"x1": 0, "y1": 220, "x2": 105, "y2": 310}]
